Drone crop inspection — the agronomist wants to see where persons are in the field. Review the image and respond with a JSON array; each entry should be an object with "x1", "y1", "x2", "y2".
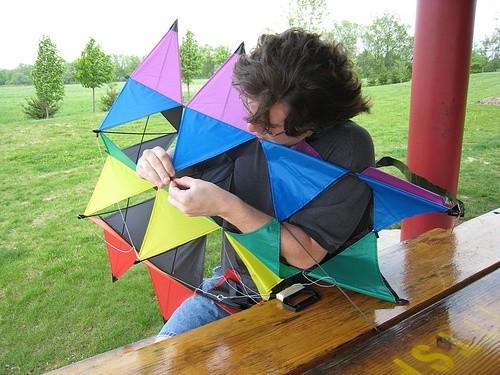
[{"x1": 135, "y1": 26, "x2": 375, "y2": 346}]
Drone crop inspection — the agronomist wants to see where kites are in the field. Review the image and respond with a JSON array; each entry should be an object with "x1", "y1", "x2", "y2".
[{"x1": 76, "y1": 18, "x2": 465, "y2": 315}]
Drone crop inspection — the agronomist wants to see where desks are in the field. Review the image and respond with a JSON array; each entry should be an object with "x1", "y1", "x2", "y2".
[{"x1": 38, "y1": 204, "x2": 500, "y2": 374}]
